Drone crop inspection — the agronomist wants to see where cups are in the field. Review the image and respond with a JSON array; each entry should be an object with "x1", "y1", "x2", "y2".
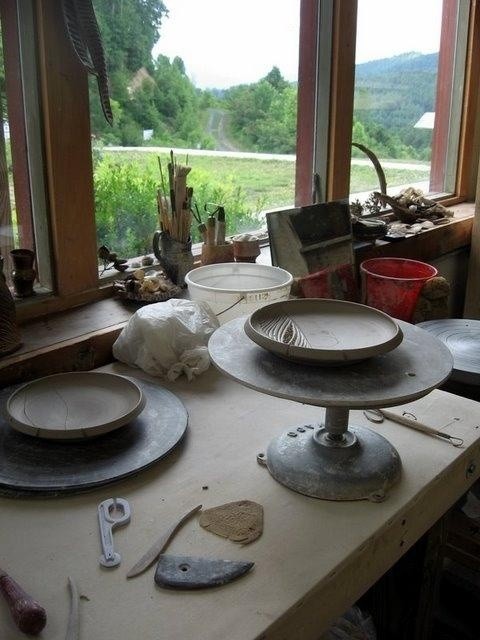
[{"x1": 152, "y1": 229, "x2": 195, "y2": 290}]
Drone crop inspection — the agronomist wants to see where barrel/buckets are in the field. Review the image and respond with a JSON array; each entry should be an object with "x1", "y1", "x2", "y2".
[
  {"x1": 297, "y1": 262, "x2": 359, "y2": 302},
  {"x1": 359, "y1": 257, "x2": 439, "y2": 322},
  {"x1": 184, "y1": 261, "x2": 293, "y2": 327}
]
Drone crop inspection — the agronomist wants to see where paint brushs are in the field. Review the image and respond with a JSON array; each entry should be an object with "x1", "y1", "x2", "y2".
[
  {"x1": 157, "y1": 150, "x2": 193, "y2": 243},
  {"x1": 366, "y1": 410, "x2": 464, "y2": 447}
]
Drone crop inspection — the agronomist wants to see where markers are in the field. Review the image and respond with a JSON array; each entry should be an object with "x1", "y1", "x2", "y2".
[{"x1": 197, "y1": 207, "x2": 227, "y2": 245}]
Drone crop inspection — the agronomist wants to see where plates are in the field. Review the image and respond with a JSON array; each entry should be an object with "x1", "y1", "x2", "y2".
[
  {"x1": 3, "y1": 371, "x2": 147, "y2": 440},
  {"x1": 244, "y1": 297, "x2": 404, "y2": 367}
]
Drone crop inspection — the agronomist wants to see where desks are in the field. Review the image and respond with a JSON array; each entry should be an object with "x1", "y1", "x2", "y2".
[{"x1": 1, "y1": 356, "x2": 480, "y2": 639}]
